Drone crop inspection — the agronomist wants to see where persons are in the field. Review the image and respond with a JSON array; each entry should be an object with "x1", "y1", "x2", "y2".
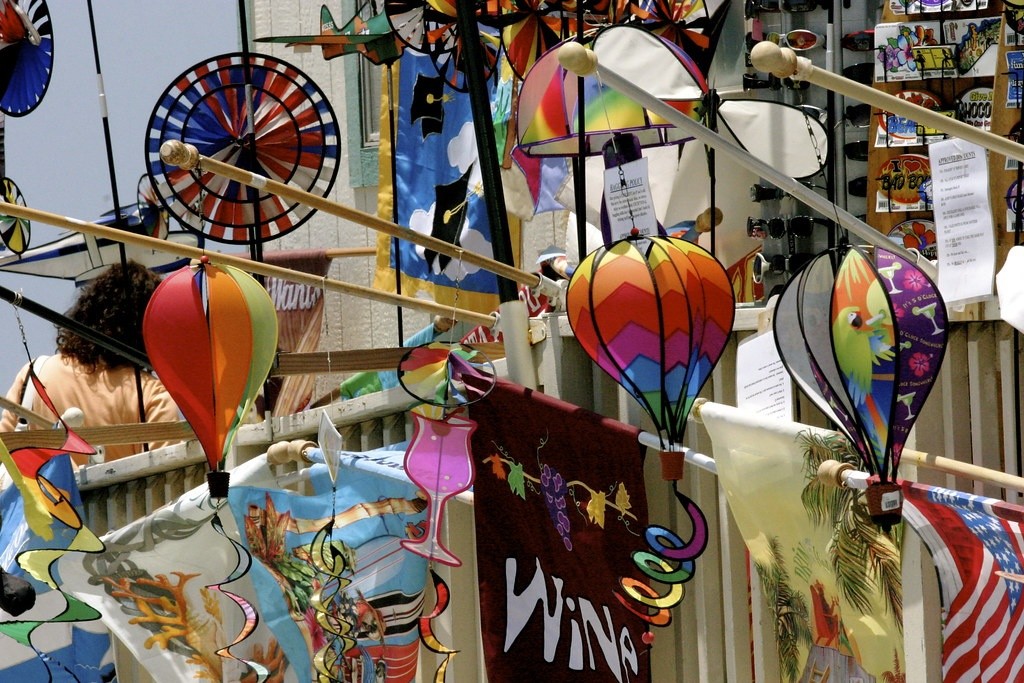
[{"x1": 1, "y1": 257, "x2": 180, "y2": 469}]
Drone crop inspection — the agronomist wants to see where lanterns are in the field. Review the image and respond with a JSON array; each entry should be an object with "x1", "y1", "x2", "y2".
[
  {"x1": 560, "y1": 223, "x2": 736, "y2": 649},
  {"x1": 771, "y1": 243, "x2": 951, "y2": 528},
  {"x1": 142, "y1": 251, "x2": 280, "y2": 512}
]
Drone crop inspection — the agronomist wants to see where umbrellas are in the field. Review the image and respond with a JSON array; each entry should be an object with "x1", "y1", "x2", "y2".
[{"x1": 517, "y1": 24, "x2": 707, "y2": 157}]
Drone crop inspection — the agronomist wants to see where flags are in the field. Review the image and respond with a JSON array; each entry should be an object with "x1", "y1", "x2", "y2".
[
  {"x1": 460, "y1": 366, "x2": 653, "y2": 682},
  {"x1": 371, "y1": 36, "x2": 521, "y2": 314},
  {"x1": 0, "y1": 433, "x2": 452, "y2": 683},
  {"x1": 699, "y1": 398, "x2": 905, "y2": 682},
  {"x1": 867, "y1": 478, "x2": 1024, "y2": 683}
]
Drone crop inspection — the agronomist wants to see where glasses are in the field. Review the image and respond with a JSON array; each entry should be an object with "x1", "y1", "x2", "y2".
[
  {"x1": 745, "y1": 0, "x2": 759, "y2": 20},
  {"x1": 844, "y1": 140, "x2": 868, "y2": 161},
  {"x1": 842, "y1": 63, "x2": 874, "y2": 88},
  {"x1": 746, "y1": 216, "x2": 766, "y2": 239},
  {"x1": 768, "y1": 216, "x2": 814, "y2": 238},
  {"x1": 771, "y1": 254, "x2": 811, "y2": 275},
  {"x1": 768, "y1": 73, "x2": 810, "y2": 91},
  {"x1": 753, "y1": 253, "x2": 774, "y2": 285},
  {"x1": 766, "y1": 30, "x2": 825, "y2": 51},
  {"x1": 848, "y1": 176, "x2": 867, "y2": 198},
  {"x1": 742, "y1": 74, "x2": 768, "y2": 91},
  {"x1": 800, "y1": 181, "x2": 827, "y2": 192},
  {"x1": 846, "y1": 104, "x2": 870, "y2": 128},
  {"x1": 746, "y1": 32, "x2": 767, "y2": 51},
  {"x1": 855, "y1": 214, "x2": 866, "y2": 222},
  {"x1": 750, "y1": 184, "x2": 769, "y2": 202},
  {"x1": 840, "y1": 30, "x2": 874, "y2": 52},
  {"x1": 745, "y1": 52, "x2": 753, "y2": 67},
  {"x1": 760, "y1": 0, "x2": 818, "y2": 12},
  {"x1": 795, "y1": 104, "x2": 827, "y2": 125}
]
